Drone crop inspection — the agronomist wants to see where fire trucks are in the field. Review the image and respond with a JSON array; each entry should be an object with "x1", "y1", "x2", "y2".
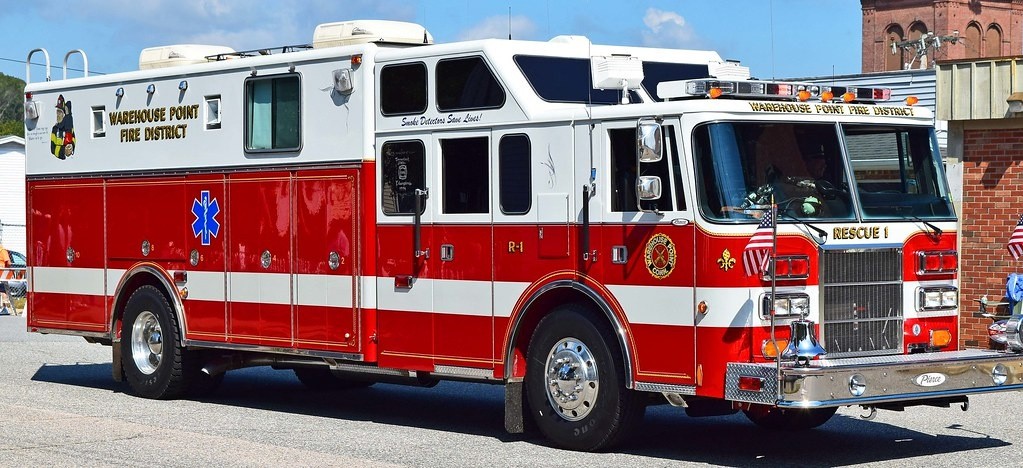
[{"x1": 22, "y1": 19, "x2": 1023, "y2": 453}]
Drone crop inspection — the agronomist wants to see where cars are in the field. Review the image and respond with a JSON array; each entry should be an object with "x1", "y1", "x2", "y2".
[{"x1": 1, "y1": 250, "x2": 27, "y2": 296}]
